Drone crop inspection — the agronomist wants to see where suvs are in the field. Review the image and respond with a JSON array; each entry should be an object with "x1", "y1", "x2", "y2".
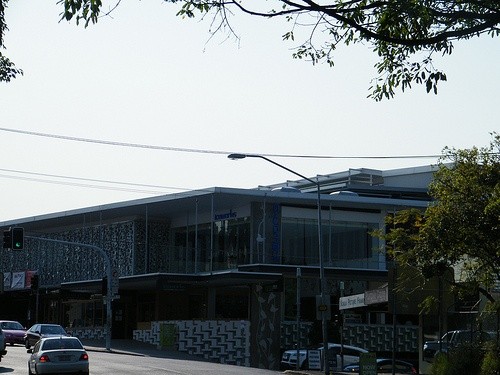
[{"x1": 280, "y1": 343, "x2": 368, "y2": 371}]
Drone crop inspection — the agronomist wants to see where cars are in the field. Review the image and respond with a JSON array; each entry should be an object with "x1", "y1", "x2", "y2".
[
  {"x1": 0, "y1": 323, "x2": 7, "y2": 362},
  {"x1": 26, "y1": 336, "x2": 90, "y2": 375},
  {"x1": 422, "y1": 330, "x2": 478, "y2": 357},
  {"x1": 343, "y1": 358, "x2": 417, "y2": 375},
  {"x1": 23, "y1": 323, "x2": 70, "y2": 349},
  {"x1": 0, "y1": 319, "x2": 28, "y2": 346}
]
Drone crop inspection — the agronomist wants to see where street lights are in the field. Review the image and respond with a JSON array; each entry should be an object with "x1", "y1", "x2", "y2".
[{"x1": 227, "y1": 153, "x2": 332, "y2": 375}]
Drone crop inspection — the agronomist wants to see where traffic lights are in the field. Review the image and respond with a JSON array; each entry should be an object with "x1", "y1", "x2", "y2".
[
  {"x1": 12, "y1": 227, "x2": 25, "y2": 251},
  {"x1": 3, "y1": 227, "x2": 13, "y2": 249},
  {"x1": 30, "y1": 274, "x2": 39, "y2": 291}
]
